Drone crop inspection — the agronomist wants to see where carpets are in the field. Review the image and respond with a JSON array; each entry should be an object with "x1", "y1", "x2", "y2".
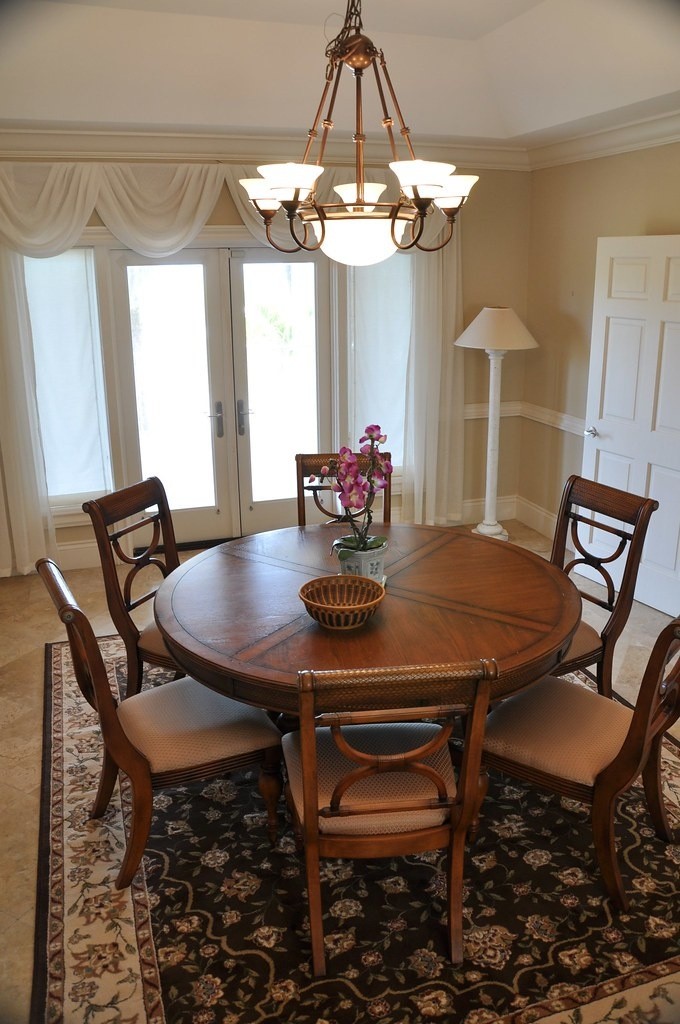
[{"x1": 29, "y1": 632, "x2": 680, "y2": 1024}]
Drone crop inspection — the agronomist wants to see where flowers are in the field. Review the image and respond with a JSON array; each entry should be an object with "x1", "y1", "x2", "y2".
[{"x1": 308, "y1": 424, "x2": 401, "y2": 561}]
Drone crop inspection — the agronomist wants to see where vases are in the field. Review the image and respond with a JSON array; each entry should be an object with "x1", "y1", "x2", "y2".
[{"x1": 335, "y1": 541, "x2": 387, "y2": 587}]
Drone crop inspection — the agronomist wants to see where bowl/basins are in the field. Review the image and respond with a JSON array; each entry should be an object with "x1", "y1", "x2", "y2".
[{"x1": 298, "y1": 575, "x2": 385, "y2": 630}]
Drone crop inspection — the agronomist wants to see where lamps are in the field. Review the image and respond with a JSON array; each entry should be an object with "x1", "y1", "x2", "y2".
[
  {"x1": 454, "y1": 307, "x2": 539, "y2": 541},
  {"x1": 239, "y1": 0, "x2": 481, "y2": 267}
]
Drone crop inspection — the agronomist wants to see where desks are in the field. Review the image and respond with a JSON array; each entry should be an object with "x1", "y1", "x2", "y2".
[{"x1": 154, "y1": 522, "x2": 583, "y2": 717}]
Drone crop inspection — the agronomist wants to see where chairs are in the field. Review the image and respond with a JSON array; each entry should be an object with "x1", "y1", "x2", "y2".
[
  {"x1": 281, "y1": 660, "x2": 500, "y2": 975},
  {"x1": 81, "y1": 476, "x2": 190, "y2": 699},
  {"x1": 460, "y1": 614, "x2": 680, "y2": 912},
  {"x1": 35, "y1": 557, "x2": 285, "y2": 889},
  {"x1": 462, "y1": 474, "x2": 659, "y2": 736},
  {"x1": 296, "y1": 452, "x2": 392, "y2": 526}
]
